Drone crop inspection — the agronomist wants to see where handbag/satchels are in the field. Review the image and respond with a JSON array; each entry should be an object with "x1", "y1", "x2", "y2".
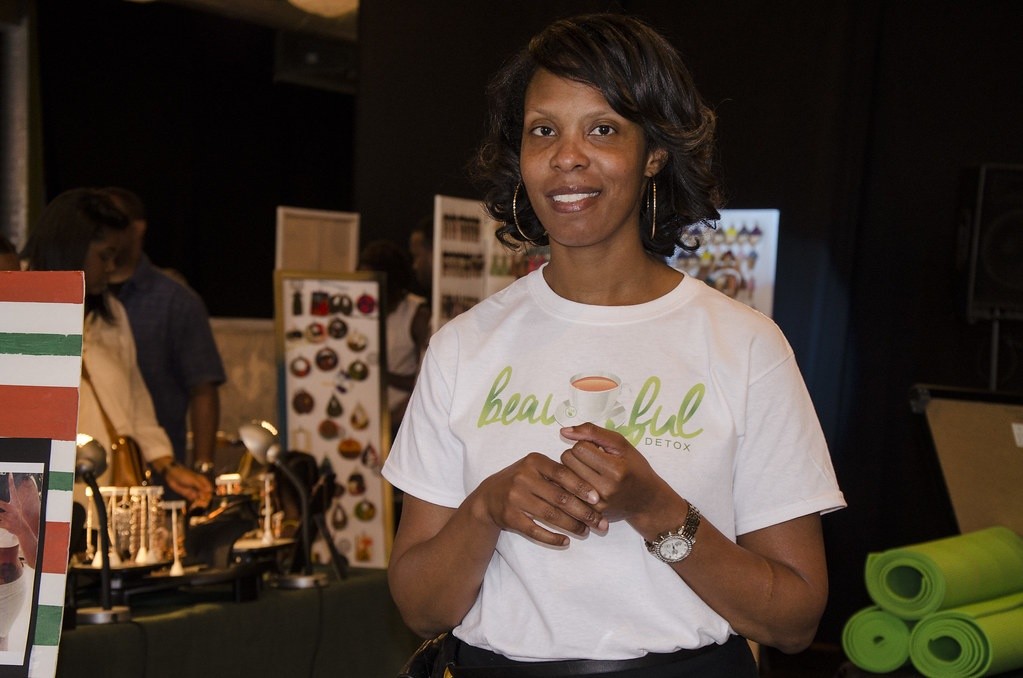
[{"x1": 110, "y1": 435, "x2": 150, "y2": 487}]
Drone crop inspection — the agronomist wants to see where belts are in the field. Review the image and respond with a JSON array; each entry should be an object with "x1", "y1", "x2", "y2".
[{"x1": 457, "y1": 633, "x2": 743, "y2": 678}]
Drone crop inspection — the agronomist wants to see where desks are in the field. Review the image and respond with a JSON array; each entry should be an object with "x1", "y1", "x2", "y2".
[{"x1": 56, "y1": 563, "x2": 423, "y2": 677}]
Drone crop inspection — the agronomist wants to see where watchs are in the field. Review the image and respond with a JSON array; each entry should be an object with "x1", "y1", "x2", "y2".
[
  {"x1": 192, "y1": 460, "x2": 215, "y2": 479},
  {"x1": 644, "y1": 498, "x2": 700, "y2": 564}
]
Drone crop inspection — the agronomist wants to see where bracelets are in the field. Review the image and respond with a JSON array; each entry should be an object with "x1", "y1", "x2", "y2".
[{"x1": 160, "y1": 461, "x2": 179, "y2": 479}]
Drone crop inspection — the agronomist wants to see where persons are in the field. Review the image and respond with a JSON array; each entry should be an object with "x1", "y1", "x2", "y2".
[
  {"x1": 0, "y1": 187, "x2": 227, "y2": 519},
  {"x1": 0, "y1": 473, "x2": 41, "y2": 650},
  {"x1": 380, "y1": 13, "x2": 848, "y2": 678},
  {"x1": 360, "y1": 214, "x2": 434, "y2": 427}
]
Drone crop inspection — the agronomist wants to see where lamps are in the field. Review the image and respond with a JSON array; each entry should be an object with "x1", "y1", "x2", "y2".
[
  {"x1": 74, "y1": 431, "x2": 131, "y2": 624},
  {"x1": 234, "y1": 419, "x2": 327, "y2": 589}
]
